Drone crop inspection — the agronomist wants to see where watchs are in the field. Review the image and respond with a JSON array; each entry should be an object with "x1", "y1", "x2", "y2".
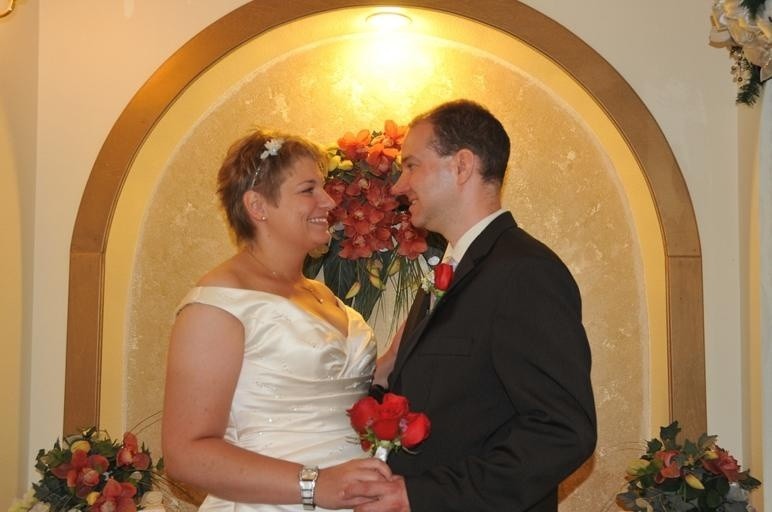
[{"x1": 298, "y1": 465, "x2": 319, "y2": 510}]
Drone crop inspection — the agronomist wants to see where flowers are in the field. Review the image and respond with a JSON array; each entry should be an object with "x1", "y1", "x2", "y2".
[
  {"x1": 615, "y1": 418, "x2": 762, "y2": 512},
  {"x1": 306, "y1": 118, "x2": 447, "y2": 343},
  {"x1": 419, "y1": 261, "x2": 454, "y2": 302},
  {"x1": 5, "y1": 428, "x2": 200, "y2": 511},
  {"x1": 708, "y1": 0, "x2": 771, "y2": 108},
  {"x1": 347, "y1": 388, "x2": 431, "y2": 459}
]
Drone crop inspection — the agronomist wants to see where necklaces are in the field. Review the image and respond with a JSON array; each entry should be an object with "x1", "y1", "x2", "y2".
[{"x1": 250, "y1": 251, "x2": 324, "y2": 303}]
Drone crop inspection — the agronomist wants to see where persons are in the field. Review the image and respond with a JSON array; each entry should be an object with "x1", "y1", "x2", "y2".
[
  {"x1": 336, "y1": 100, "x2": 597, "y2": 512},
  {"x1": 162, "y1": 127, "x2": 394, "y2": 512}
]
